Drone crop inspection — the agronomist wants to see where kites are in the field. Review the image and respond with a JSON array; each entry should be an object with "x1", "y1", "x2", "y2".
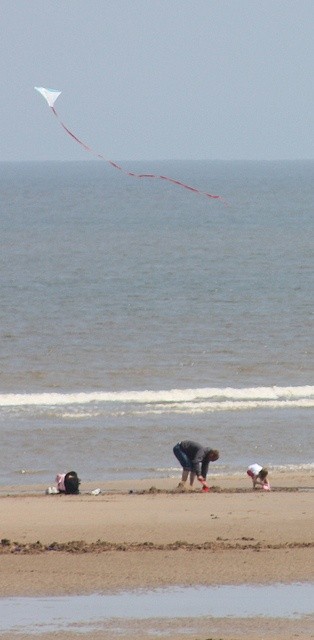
[{"x1": 35, "y1": 86, "x2": 219, "y2": 199}]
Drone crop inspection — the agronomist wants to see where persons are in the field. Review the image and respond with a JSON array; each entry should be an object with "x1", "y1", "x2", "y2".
[
  {"x1": 247, "y1": 463, "x2": 269, "y2": 489},
  {"x1": 172, "y1": 440, "x2": 219, "y2": 489}
]
